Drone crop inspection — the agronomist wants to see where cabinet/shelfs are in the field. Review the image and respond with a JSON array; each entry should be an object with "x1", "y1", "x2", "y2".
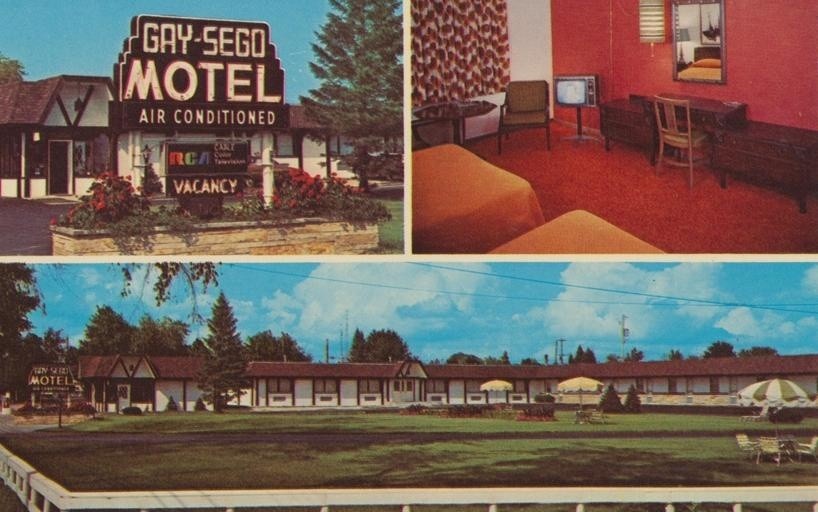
[
  {"x1": 714, "y1": 120, "x2": 818, "y2": 214},
  {"x1": 599, "y1": 98, "x2": 658, "y2": 168}
]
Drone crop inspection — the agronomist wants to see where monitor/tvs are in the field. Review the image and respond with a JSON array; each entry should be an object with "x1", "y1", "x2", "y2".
[{"x1": 555, "y1": 76, "x2": 596, "y2": 107}]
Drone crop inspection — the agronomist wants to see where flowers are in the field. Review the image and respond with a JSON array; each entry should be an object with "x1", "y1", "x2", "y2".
[{"x1": 46, "y1": 165, "x2": 393, "y2": 238}]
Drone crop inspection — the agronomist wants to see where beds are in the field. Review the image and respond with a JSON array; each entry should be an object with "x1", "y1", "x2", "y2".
[
  {"x1": 415, "y1": 100, "x2": 499, "y2": 147},
  {"x1": 412, "y1": 142, "x2": 665, "y2": 254},
  {"x1": 678, "y1": 47, "x2": 721, "y2": 80}
]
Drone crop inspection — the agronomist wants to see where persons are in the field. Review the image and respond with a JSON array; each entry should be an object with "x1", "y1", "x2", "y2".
[{"x1": 559, "y1": 83, "x2": 585, "y2": 104}]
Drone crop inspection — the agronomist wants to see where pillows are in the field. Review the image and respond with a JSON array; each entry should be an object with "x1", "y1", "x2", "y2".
[{"x1": 692, "y1": 58, "x2": 721, "y2": 68}]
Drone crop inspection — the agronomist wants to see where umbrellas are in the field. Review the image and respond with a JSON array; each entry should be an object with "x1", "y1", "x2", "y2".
[
  {"x1": 480, "y1": 378, "x2": 513, "y2": 404},
  {"x1": 736, "y1": 378, "x2": 814, "y2": 439},
  {"x1": 556, "y1": 375, "x2": 604, "y2": 411}
]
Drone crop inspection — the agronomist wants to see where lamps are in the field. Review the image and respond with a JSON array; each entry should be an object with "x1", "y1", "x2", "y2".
[
  {"x1": 638, "y1": 0, "x2": 666, "y2": 58},
  {"x1": 676, "y1": 28, "x2": 690, "y2": 64}
]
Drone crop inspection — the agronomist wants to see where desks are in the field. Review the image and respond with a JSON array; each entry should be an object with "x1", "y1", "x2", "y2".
[{"x1": 642, "y1": 93, "x2": 748, "y2": 167}]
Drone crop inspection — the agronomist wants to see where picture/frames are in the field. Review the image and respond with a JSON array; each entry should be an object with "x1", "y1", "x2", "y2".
[{"x1": 699, "y1": 4, "x2": 721, "y2": 46}]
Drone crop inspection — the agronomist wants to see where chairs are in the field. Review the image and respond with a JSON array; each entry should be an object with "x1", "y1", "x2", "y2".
[
  {"x1": 496, "y1": 79, "x2": 551, "y2": 155},
  {"x1": 733, "y1": 431, "x2": 818, "y2": 465},
  {"x1": 652, "y1": 94, "x2": 712, "y2": 190}
]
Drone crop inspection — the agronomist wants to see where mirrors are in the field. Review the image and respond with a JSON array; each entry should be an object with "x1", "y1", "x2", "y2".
[{"x1": 671, "y1": 1, "x2": 727, "y2": 85}]
